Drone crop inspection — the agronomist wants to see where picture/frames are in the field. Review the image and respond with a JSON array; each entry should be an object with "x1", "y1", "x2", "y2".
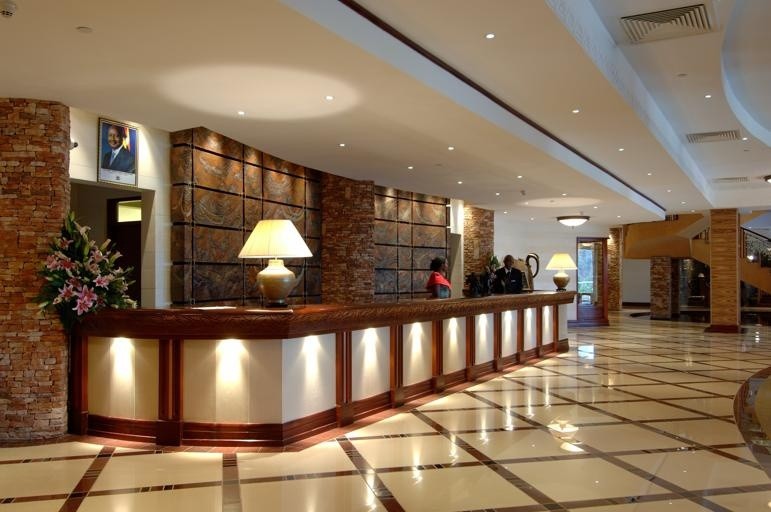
[{"x1": 96, "y1": 116, "x2": 140, "y2": 192}]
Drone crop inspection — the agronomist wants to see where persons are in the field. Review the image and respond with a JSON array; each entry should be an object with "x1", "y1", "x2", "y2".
[
  {"x1": 101, "y1": 124, "x2": 134, "y2": 173},
  {"x1": 493, "y1": 255, "x2": 522, "y2": 294},
  {"x1": 426, "y1": 256, "x2": 450, "y2": 298}
]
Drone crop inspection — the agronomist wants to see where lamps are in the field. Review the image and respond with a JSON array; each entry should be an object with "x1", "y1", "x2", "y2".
[
  {"x1": 545, "y1": 253, "x2": 578, "y2": 292},
  {"x1": 237, "y1": 218, "x2": 313, "y2": 309},
  {"x1": 556, "y1": 216, "x2": 591, "y2": 228},
  {"x1": 546, "y1": 419, "x2": 579, "y2": 440}
]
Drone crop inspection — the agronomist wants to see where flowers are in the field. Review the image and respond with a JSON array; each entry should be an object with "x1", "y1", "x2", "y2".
[{"x1": 30, "y1": 209, "x2": 139, "y2": 337}]
